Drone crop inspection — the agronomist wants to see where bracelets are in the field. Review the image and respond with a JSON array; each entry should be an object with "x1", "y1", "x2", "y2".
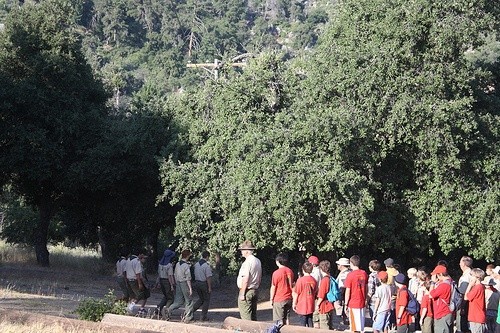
[
  {"x1": 373, "y1": 314, "x2": 377, "y2": 316},
  {"x1": 344, "y1": 303, "x2": 348, "y2": 306},
  {"x1": 429, "y1": 281, "x2": 435, "y2": 285},
  {"x1": 365, "y1": 298, "x2": 368, "y2": 301},
  {"x1": 397, "y1": 318, "x2": 401, "y2": 319},
  {"x1": 270, "y1": 298, "x2": 273, "y2": 300}
]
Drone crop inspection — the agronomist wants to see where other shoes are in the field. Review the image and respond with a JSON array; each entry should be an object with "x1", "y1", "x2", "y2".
[
  {"x1": 154, "y1": 308, "x2": 163, "y2": 317},
  {"x1": 129, "y1": 304, "x2": 136, "y2": 310},
  {"x1": 138, "y1": 308, "x2": 146, "y2": 313},
  {"x1": 165, "y1": 309, "x2": 170, "y2": 320}
]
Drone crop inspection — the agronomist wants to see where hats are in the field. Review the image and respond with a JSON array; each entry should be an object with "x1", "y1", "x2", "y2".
[
  {"x1": 393, "y1": 273, "x2": 407, "y2": 284},
  {"x1": 160, "y1": 250, "x2": 175, "y2": 265},
  {"x1": 376, "y1": 271, "x2": 388, "y2": 279},
  {"x1": 238, "y1": 240, "x2": 256, "y2": 249},
  {"x1": 492, "y1": 266, "x2": 500, "y2": 274},
  {"x1": 308, "y1": 256, "x2": 319, "y2": 265},
  {"x1": 384, "y1": 258, "x2": 393, "y2": 265},
  {"x1": 481, "y1": 276, "x2": 496, "y2": 285},
  {"x1": 335, "y1": 258, "x2": 350, "y2": 265},
  {"x1": 431, "y1": 265, "x2": 446, "y2": 276}
]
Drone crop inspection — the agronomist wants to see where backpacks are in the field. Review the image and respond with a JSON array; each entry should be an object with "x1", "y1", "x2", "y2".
[
  {"x1": 403, "y1": 288, "x2": 420, "y2": 315},
  {"x1": 325, "y1": 276, "x2": 342, "y2": 302},
  {"x1": 442, "y1": 281, "x2": 466, "y2": 312}
]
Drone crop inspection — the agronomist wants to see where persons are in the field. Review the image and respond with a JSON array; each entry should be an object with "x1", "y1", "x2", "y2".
[
  {"x1": 429, "y1": 264, "x2": 456, "y2": 333},
  {"x1": 372, "y1": 271, "x2": 392, "y2": 333},
  {"x1": 458, "y1": 255, "x2": 474, "y2": 333},
  {"x1": 364, "y1": 258, "x2": 402, "y2": 333},
  {"x1": 334, "y1": 258, "x2": 352, "y2": 329},
  {"x1": 115, "y1": 247, "x2": 150, "y2": 314},
  {"x1": 483, "y1": 265, "x2": 500, "y2": 333},
  {"x1": 153, "y1": 250, "x2": 174, "y2": 319},
  {"x1": 180, "y1": 251, "x2": 212, "y2": 322},
  {"x1": 394, "y1": 273, "x2": 415, "y2": 333},
  {"x1": 315, "y1": 260, "x2": 334, "y2": 329},
  {"x1": 308, "y1": 256, "x2": 321, "y2": 323},
  {"x1": 292, "y1": 262, "x2": 318, "y2": 328},
  {"x1": 269, "y1": 253, "x2": 294, "y2": 326},
  {"x1": 344, "y1": 255, "x2": 367, "y2": 333},
  {"x1": 237, "y1": 242, "x2": 262, "y2": 321},
  {"x1": 464, "y1": 266, "x2": 486, "y2": 333},
  {"x1": 407, "y1": 258, "x2": 454, "y2": 333},
  {"x1": 161, "y1": 250, "x2": 199, "y2": 322}
]
